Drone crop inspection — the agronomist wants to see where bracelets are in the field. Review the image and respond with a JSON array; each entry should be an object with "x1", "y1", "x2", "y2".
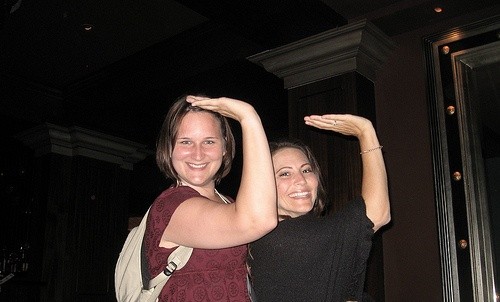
[{"x1": 359, "y1": 146, "x2": 383, "y2": 155}]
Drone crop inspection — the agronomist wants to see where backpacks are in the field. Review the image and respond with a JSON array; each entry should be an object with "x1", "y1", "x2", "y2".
[{"x1": 113, "y1": 201, "x2": 195, "y2": 301}]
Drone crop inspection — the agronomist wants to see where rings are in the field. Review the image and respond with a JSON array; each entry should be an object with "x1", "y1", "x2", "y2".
[{"x1": 334, "y1": 120, "x2": 336, "y2": 127}]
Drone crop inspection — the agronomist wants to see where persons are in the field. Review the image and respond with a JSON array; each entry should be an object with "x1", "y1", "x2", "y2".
[
  {"x1": 141, "y1": 94, "x2": 278, "y2": 302},
  {"x1": 251, "y1": 113, "x2": 391, "y2": 302}
]
